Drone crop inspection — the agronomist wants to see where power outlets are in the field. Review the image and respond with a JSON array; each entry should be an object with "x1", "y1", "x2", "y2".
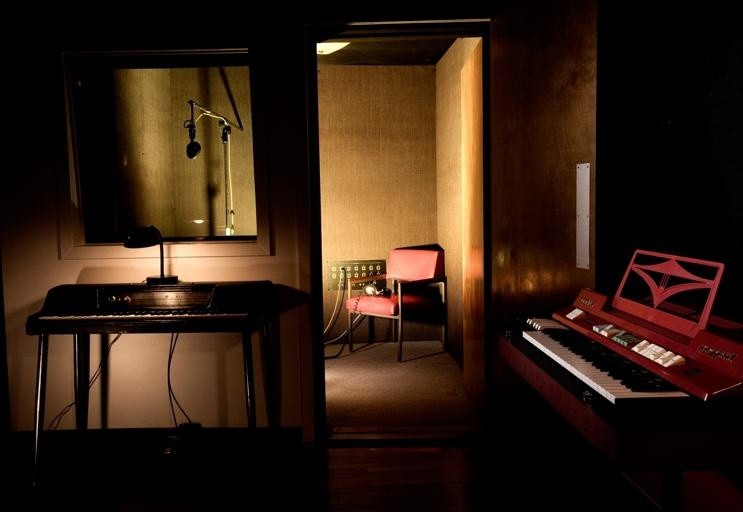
[{"x1": 326, "y1": 259, "x2": 386, "y2": 293}]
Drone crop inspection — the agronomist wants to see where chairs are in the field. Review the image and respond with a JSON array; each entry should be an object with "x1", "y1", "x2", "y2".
[{"x1": 345, "y1": 245, "x2": 449, "y2": 364}]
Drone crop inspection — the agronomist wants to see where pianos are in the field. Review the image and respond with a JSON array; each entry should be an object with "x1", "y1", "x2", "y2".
[
  {"x1": 496, "y1": 287, "x2": 741, "y2": 454},
  {"x1": 25, "y1": 280, "x2": 275, "y2": 333}
]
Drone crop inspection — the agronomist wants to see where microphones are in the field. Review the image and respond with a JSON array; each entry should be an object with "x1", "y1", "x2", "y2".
[{"x1": 186, "y1": 129, "x2": 201, "y2": 159}]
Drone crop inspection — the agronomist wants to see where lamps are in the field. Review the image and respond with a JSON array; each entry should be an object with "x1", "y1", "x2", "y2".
[{"x1": 120, "y1": 223, "x2": 179, "y2": 284}]
